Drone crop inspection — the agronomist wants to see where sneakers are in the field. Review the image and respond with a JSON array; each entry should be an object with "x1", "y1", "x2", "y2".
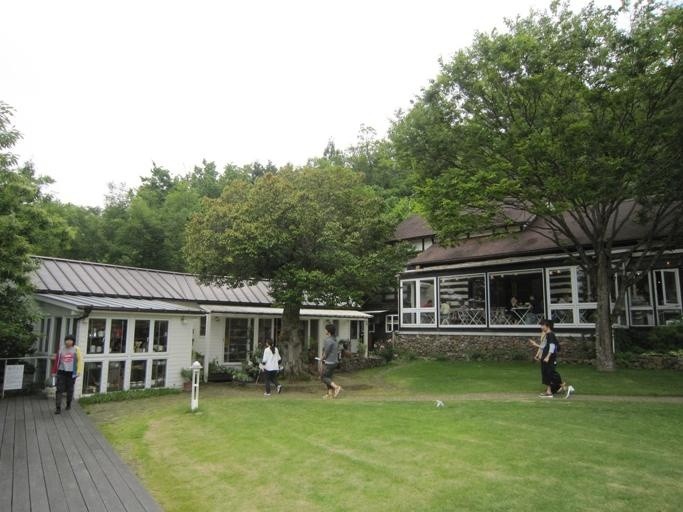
[
  {"x1": 538, "y1": 382, "x2": 575, "y2": 399},
  {"x1": 55, "y1": 404, "x2": 71, "y2": 414},
  {"x1": 322, "y1": 386, "x2": 340, "y2": 400},
  {"x1": 264, "y1": 384, "x2": 282, "y2": 396}
]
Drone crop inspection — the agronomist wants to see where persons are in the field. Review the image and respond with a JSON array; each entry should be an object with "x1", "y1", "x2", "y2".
[
  {"x1": 523, "y1": 294, "x2": 543, "y2": 324},
  {"x1": 533, "y1": 319, "x2": 568, "y2": 400},
  {"x1": 261, "y1": 336, "x2": 283, "y2": 396},
  {"x1": 504, "y1": 296, "x2": 522, "y2": 325},
  {"x1": 439, "y1": 299, "x2": 450, "y2": 324},
  {"x1": 318, "y1": 323, "x2": 343, "y2": 400},
  {"x1": 527, "y1": 320, "x2": 562, "y2": 394},
  {"x1": 557, "y1": 294, "x2": 571, "y2": 323},
  {"x1": 50, "y1": 333, "x2": 82, "y2": 416},
  {"x1": 419, "y1": 299, "x2": 434, "y2": 324}
]
